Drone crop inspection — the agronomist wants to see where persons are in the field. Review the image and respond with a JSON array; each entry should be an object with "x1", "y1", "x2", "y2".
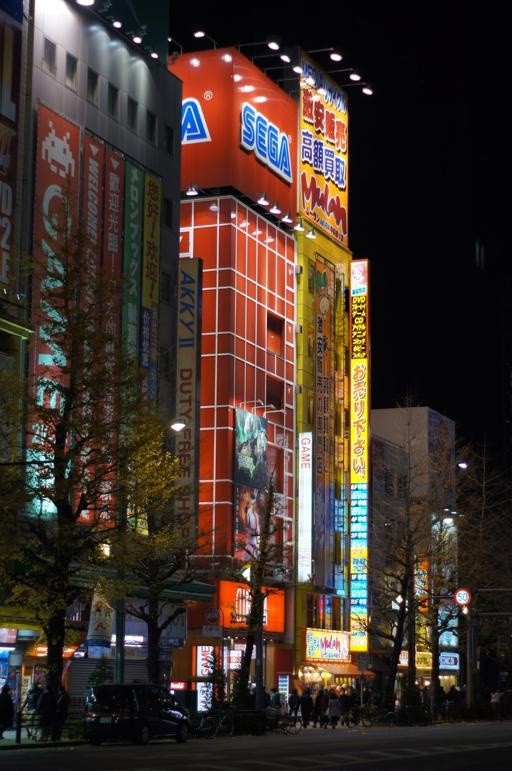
[
  {"x1": 269, "y1": 686, "x2": 358, "y2": 731},
  {"x1": 416, "y1": 685, "x2": 506, "y2": 724},
  {"x1": 1, "y1": 681, "x2": 70, "y2": 743}
]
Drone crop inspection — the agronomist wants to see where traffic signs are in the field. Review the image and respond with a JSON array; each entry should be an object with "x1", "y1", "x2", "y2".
[{"x1": 454, "y1": 587, "x2": 472, "y2": 606}]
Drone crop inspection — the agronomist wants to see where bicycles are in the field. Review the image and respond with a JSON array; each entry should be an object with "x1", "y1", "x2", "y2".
[
  {"x1": 343, "y1": 705, "x2": 373, "y2": 729},
  {"x1": 262, "y1": 711, "x2": 302, "y2": 735},
  {"x1": 192, "y1": 709, "x2": 235, "y2": 739}
]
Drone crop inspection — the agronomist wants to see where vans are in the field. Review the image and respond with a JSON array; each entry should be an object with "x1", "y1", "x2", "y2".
[{"x1": 82, "y1": 684, "x2": 194, "y2": 747}]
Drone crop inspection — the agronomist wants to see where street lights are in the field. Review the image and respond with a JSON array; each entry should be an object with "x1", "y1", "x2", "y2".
[{"x1": 115, "y1": 415, "x2": 190, "y2": 685}]
